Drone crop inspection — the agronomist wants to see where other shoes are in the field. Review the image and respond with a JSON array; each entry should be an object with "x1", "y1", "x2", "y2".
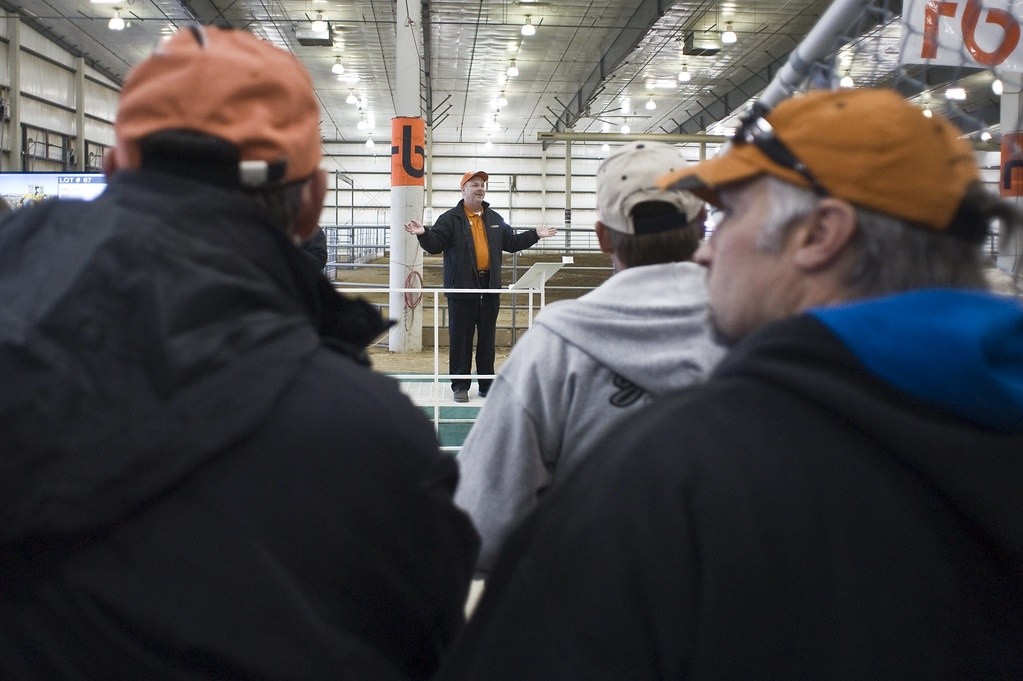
[
  {"x1": 478, "y1": 389, "x2": 487, "y2": 396},
  {"x1": 454, "y1": 388, "x2": 469, "y2": 402}
]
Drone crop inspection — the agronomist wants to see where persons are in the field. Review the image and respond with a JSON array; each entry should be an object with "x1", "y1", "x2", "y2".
[
  {"x1": 405, "y1": 170, "x2": 557, "y2": 402},
  {"x1": 440, "y1": 86, "x2": 1023, "y2": 681},
  {"x1": 2, "y1": 27, "x2": 481, "y2": 680},
  {"x1": 445, "y1": 141, "x2": 729, "y2": 580},
  {"x1": 297, "y1": 224, "x2": 328, "y2": 270}
]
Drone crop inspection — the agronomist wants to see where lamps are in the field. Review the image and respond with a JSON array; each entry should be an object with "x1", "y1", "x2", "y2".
[
  {"x1": 840, "y1": 69, "x2": 856, "y2": 87},
  {"x1": 721, "y1": 24, "x2": 737, "y2": 44},
  {"x1": 647, "y1": 95, "x2": 657, "y2": 110},
  {"x1": 346, "y1": 91, "x2": 358, "y2": 103},
  {"x1": 358, "y1": 114, "x2": 370, "y2": 130},
  {"x1": 108, "y1": 10, "x2": 125, "y2": 31},
  {"x1": 332, "y1": 58, "x2": 344, "y2": 76},
  {"x1": 521, "y1": 18, "x2": 535, "y2": 36},
  {"x1": 311, "y1": 13, "x2": 326, "y2": 32},
  {"x1": 622, "y1": 118, "x2": 631, "y2": 133},
  {"x1": 923, "y1": 102, "x2": 935, "y2": 120},
  {"x1": 498, "y1": 94, "x2": 509, "y2": 107},
  {"x1": 679, "y1": 65, "x2": 691, "y2": 80},
  {"x1": 365, "y1": 136, "x2": 375, "y2": 149},
  {"x1": 507, "y1": 61, "x2": 518, "y2": 77}
]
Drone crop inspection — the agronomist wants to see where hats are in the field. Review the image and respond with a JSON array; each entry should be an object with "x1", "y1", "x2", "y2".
[
  {"x1": 657, "y1": 87, "x2": 982, "y2": 228},
  {"x1": 460, "y1": 171, "x2": 489, "y2": 186},
  {"x1": 595, "y1": 140, "x2": 705, "y2": 235},
  {"x1": 114, "y1": 25, "x2": 323, "y2": 191}
]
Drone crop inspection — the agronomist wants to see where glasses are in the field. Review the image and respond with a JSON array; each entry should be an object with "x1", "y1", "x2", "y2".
[{"x1": 730, "y1": 101, "x2": 830, "y2": 201}]
